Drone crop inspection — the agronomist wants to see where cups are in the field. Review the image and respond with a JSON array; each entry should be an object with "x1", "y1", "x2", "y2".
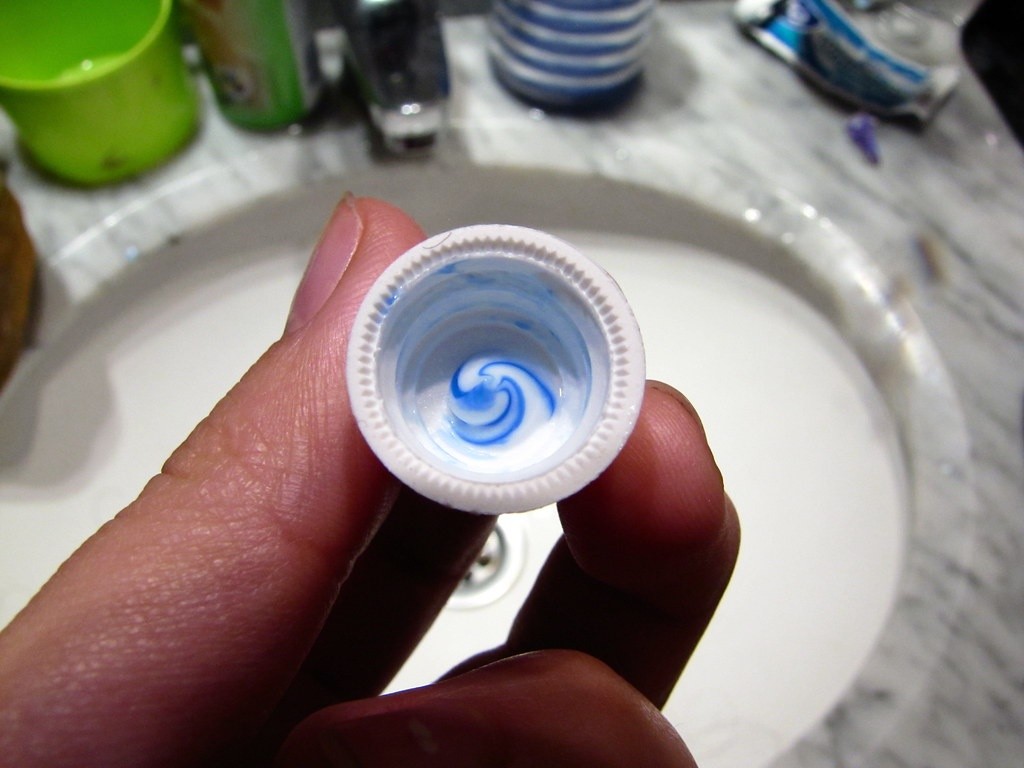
[{"x1": 0, "y1": 0, "x2": 196, "y2": 184}]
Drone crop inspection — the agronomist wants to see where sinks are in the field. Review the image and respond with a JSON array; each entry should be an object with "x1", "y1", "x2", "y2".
[{"x1": 1, "y1": 156, "x2": 916, "y2": 768}]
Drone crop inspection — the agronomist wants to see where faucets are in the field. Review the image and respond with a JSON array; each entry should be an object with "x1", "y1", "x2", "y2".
[{"x1": 342, "y1": 1, "x2": 452, "y2": 163}]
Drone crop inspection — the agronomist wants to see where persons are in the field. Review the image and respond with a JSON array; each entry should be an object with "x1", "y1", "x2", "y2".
[{"x1": 2, "y1": 186, "x2": 740, "y2": 768}]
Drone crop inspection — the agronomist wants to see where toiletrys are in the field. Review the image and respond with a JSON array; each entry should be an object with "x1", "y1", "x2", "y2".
[{"x1": 731, "y1": 0, "x2": 962, "y2": 137}]
